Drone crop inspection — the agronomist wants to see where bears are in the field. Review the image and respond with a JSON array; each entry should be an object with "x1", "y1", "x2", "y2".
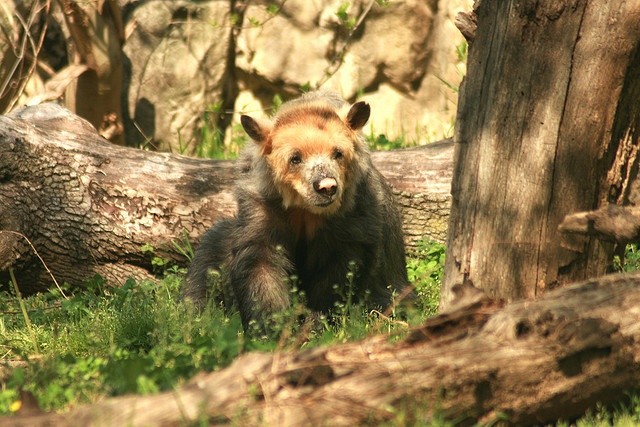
[{"x1": 176, "y1": 90, "x2": 424, "y2": 352}]
